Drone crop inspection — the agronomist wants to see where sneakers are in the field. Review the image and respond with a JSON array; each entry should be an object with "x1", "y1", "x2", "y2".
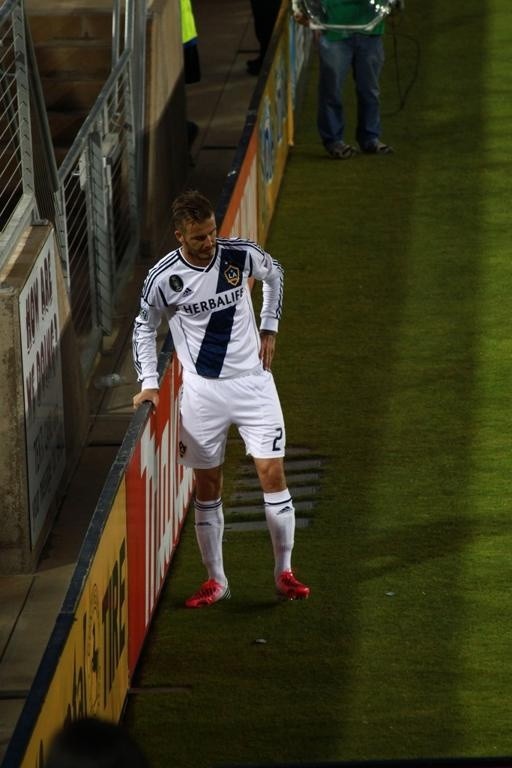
[
  {"x1": 362, "y1": 142, "x2": 393, "y2": 154},
  {"x1": 273, "y1": 571, "x2": 310, "y2": 599},
  {"x1": 329, "y1": 144, "x2": 356, "y2": 159},
  {"x1": 185, "y1": 581, "x2": 231, "y2": 608}
]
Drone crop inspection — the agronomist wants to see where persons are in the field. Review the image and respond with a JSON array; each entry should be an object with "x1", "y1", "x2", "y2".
[
  {"x1": 290, "y1": 0, "x2": 397, "y2": 159},
  {"x1": 126, "y1": 188, "x2": 314, "y2": 608},
  {"x1": 244, "y1": 1, "x2": 284, "y2": 77}
]
